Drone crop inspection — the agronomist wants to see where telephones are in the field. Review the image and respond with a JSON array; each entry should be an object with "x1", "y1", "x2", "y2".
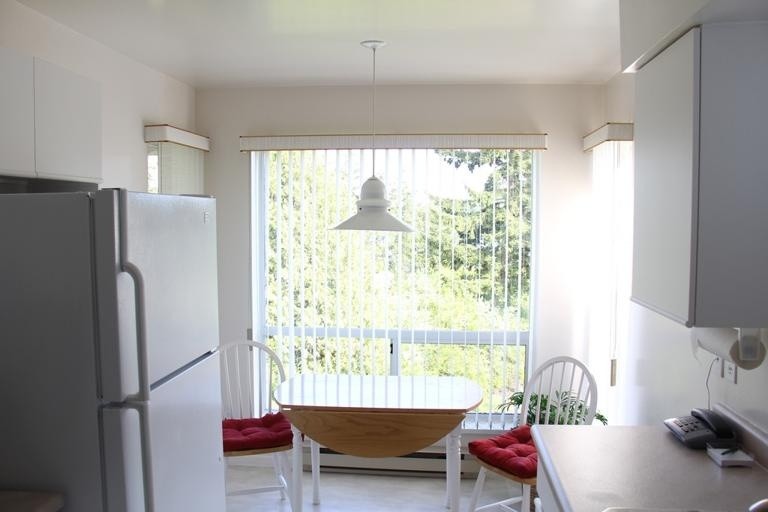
[{"x1": 664, "y1": 407, "x2": 737, "y2": 450}]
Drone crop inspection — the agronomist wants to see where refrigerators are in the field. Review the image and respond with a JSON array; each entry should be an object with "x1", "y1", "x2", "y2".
[{"x1": 7, "y1": 184, "x2": 226, "y2": 509}]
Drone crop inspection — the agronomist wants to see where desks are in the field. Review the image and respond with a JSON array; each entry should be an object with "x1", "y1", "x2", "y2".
[
  {"x1": 529, "y1": 422, "x2": 768, "y2": 511},
  {"x1": 274, "y1": 368, "x2": 483, "y2": 511}
]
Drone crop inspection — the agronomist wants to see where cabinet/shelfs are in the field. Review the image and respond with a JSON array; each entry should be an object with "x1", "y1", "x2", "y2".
[{"x1": 628, "y1": 24, "x2": 768, "y2": 331}]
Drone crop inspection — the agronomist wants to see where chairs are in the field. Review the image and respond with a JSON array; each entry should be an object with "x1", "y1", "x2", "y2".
[
  {"x1": 218, "y1": 339, "x2": 294, "y2": 512},
  {"x1": 469, "y1": 353, "x2": 598, "y2": 512}
]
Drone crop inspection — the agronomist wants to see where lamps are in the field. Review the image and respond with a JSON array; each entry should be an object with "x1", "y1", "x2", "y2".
[{"x1": 324, "y1": 40, "x2": 416, "y2": 232}]
